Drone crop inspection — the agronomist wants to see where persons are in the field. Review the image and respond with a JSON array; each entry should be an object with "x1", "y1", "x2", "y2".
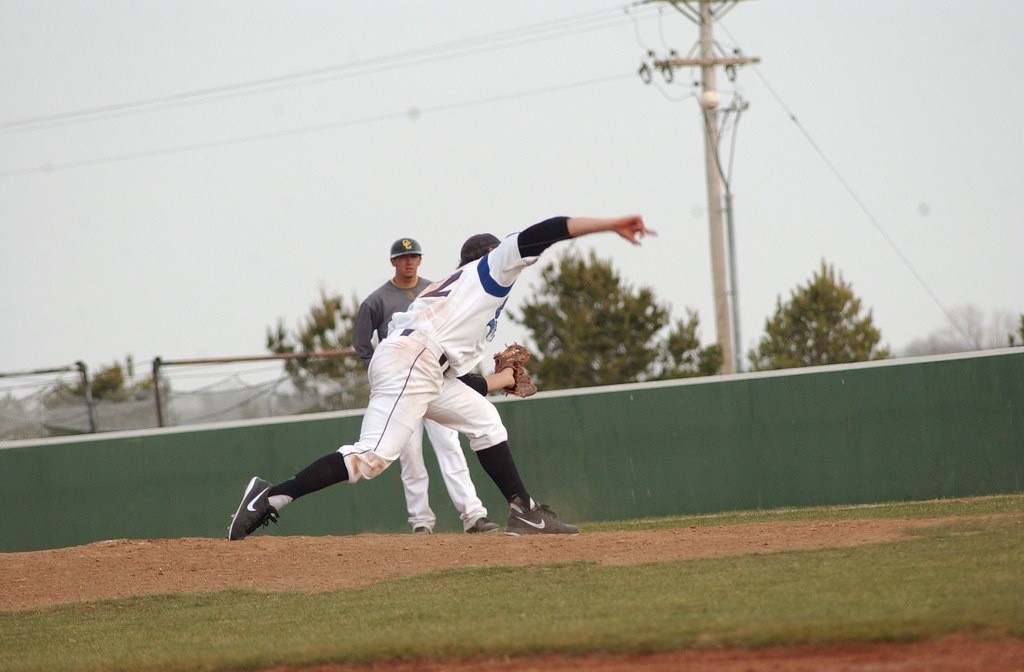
[
  {"x1": 351, "y1": 235, "x2": 498, "y2": 534},
  {"x1": 224, "y1": 213, "x2": 658, "y2": 544}
]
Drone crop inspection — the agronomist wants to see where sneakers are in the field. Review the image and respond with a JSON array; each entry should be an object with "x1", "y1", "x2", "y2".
[
  {"x1": 225, "y1": 475, "x2": 273, "y2": 542},
  {"x1": 503, "y1": 500, "x2": 581, "y2": 537},
  {"x1": 465, "y1": 516, "x2": 500, "y2": 534},
  {"x1": 412, "y1": 526, "x2": 431, "y2": 535}
]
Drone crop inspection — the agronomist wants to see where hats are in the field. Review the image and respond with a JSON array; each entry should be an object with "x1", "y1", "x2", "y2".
[
  {"x1": 389, "y1": 238, "x2": 423, "y2": 261},
  {"x1": 456, "y1": 233, "x2": 501, "y2": 269}
]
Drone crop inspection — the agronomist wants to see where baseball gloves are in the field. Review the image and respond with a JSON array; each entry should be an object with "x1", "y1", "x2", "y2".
[{"x1": 494, "y1": 343, "x2": 538, "y2": 397}]
